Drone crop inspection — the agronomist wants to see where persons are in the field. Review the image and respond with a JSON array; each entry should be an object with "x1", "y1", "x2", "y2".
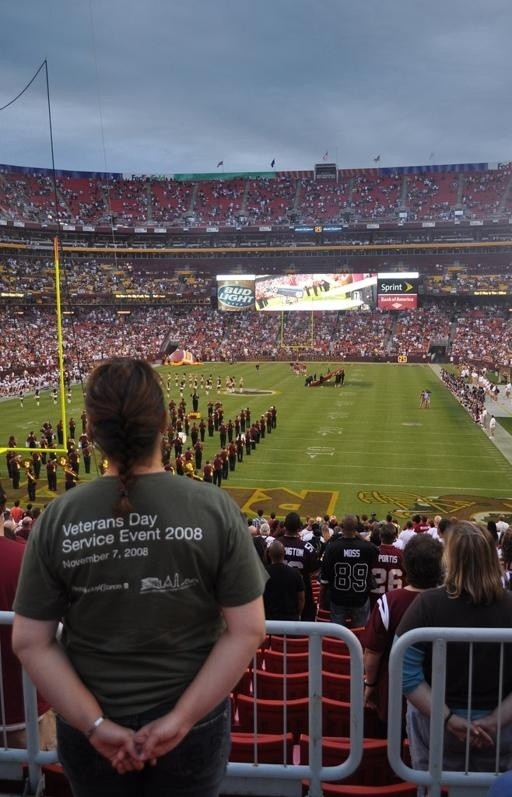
[
  {"x1": 461, "y1": 398, "x2": 495, "y2": 439},
  {"x1": 419, "y1": 389, "x2": 432, "y2": 409},
  {"x1": 1, "y1": 391, "x2": 276, "y2": 539},
  {"x1": 364, "y1": 521, "x2": 447, "y2": 736},
  {"x1": 395, "y1": 520, "x2": 511, "y2": 797},
  {"x1": 2, "y1": 170, "x2": 512, "y2": 399},
  {"x1": 11, "y1": 356, "x2": 270, "y2": 797},
  {"x1": 245, "y1": 510, "x2": 512, "y2": 628}
]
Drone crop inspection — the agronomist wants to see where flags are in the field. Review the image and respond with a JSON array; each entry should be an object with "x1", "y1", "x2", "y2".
[
  {"x1": 322, "y1": 152, "x2": 328, "y2": 161},
  {"x1": 271, "y1": 160, "x2": 275, "y2": 167},
  {"x1": 217, "y1": 161, "x2": 224, "y2": 167}
]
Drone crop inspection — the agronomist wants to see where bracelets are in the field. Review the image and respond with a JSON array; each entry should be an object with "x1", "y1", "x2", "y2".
[{"x1": 86, "y1": 715, "x2": 106, "y2": 741}]
